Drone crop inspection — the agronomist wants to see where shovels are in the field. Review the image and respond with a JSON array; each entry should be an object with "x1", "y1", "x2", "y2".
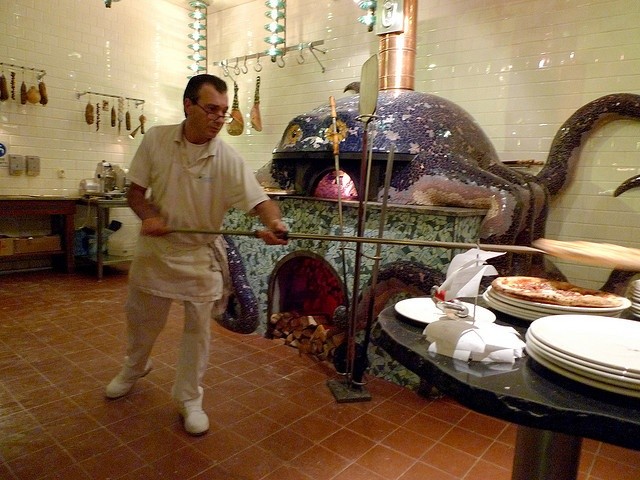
[{"x1": 346, "y1": 54, "x2": 378, "y2": 380}]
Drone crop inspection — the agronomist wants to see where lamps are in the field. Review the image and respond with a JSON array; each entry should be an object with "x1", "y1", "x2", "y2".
[
  {"x1": 185, "y1": 0, "x2": 210, "y2": 79},
  {"x1": 356, "y1": 1, "x2": 377, "y2": 33},
  {"x1": 263, "y1": 0, "x2": 287, "y2": 62}
]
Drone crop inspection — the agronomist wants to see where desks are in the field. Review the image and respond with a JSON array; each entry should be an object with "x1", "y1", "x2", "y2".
[{"x1": 1, "y1": 195, "x2": 81, "y2": 272}]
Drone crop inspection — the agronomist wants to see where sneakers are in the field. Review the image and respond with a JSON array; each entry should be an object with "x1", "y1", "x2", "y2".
[
  {"x1": 106, "y1": 360, "x2": 152, "y2": 398},
  {"x1": 181, "y1": 405, "x2": 210, "y2": 433}
]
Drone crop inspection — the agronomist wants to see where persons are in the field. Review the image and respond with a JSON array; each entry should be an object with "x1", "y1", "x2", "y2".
[{"x1": 106, "y1": 74, "x2": 290, "y2": 435}]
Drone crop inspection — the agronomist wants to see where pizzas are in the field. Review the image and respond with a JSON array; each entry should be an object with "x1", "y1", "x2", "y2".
[
  {"x1": 491, "y1": 275, "x2": 624, "y2": 309},
  {"x1": 533, "y1": 239, "x2": 640, "y2": 273}
]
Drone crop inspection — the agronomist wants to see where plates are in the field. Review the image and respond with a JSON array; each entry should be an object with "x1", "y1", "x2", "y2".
[
  {"x1": 394, "y1": 296, "x2": 496, "y2": 325},
  {"x1": 524, "y1": 313, "x2": 640, "y2": 397},
  {"x1": 632, "y1": 282, "x2": 640, "y2": 318},
  {"x1": 481, "y1": 291, "x2": 632, "y2": 321}
]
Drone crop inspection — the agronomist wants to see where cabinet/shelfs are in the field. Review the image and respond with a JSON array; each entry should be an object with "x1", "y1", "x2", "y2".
[{"x1": 85, "y1": 193, "x2": 137, "y2": 282}]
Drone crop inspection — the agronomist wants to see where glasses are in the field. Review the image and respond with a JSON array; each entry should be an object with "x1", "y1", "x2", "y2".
[{"x1": 196, "y1": 103, "x2": 234, "y2": 124}]
